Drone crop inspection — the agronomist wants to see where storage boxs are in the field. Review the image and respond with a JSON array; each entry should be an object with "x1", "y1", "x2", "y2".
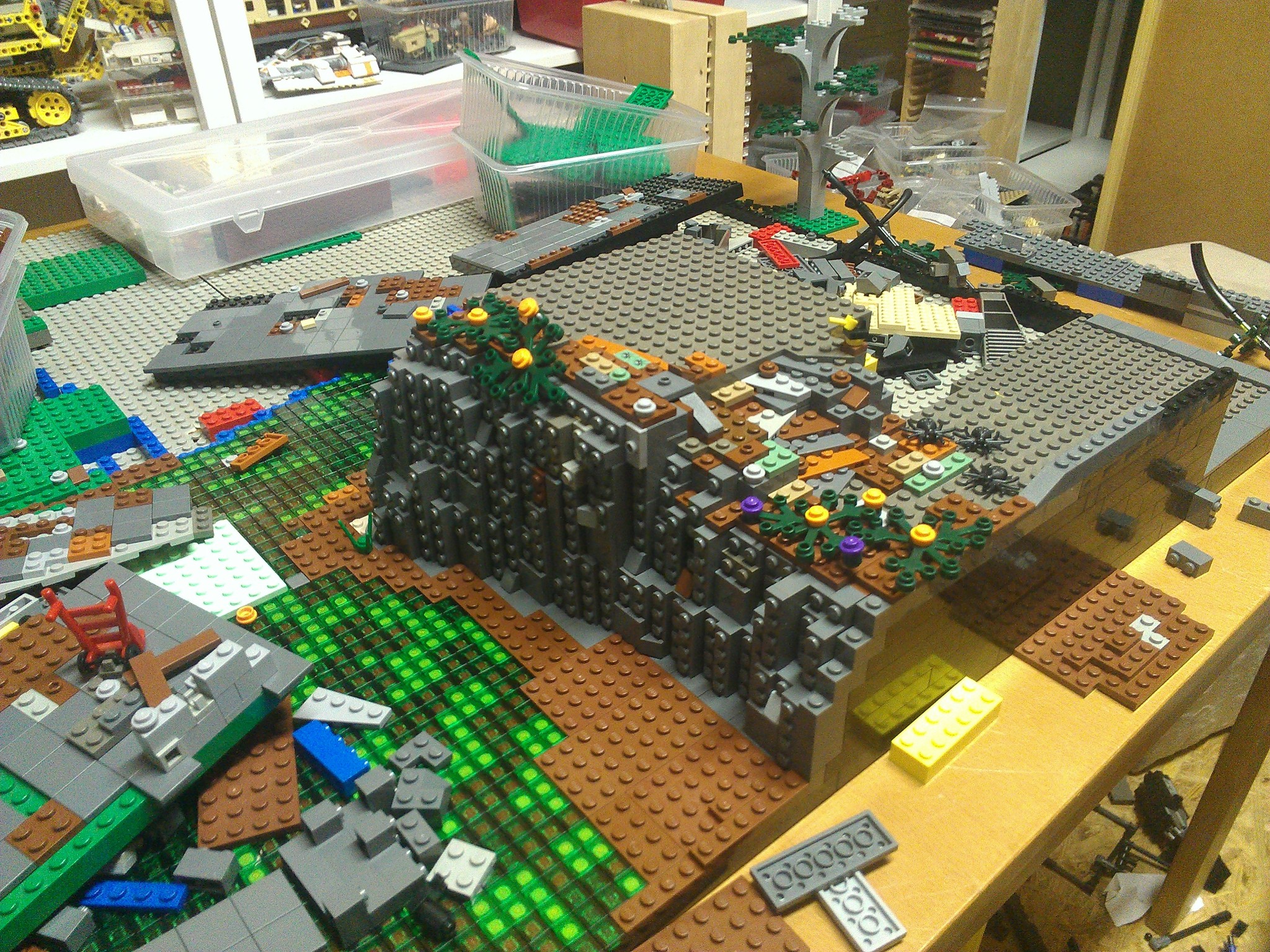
[
  {"x1": 454, "y1": 50, "x2": 714, "y2": 166},
  {"x1": 0, "y1": 208, "x2": 38, "y2": 452},
  {"x1": 66, "y1": 79, "x2": 475, "y2": 280},
  {"x1": 356, "y1": 0, "x2": 515, "y2": 64},
  {"x1": 747, "y1": 47, "x2": 1083, "y2": 240},
  {"x1": 452, "y1": 124, "x2": 711, "y2": 231}
]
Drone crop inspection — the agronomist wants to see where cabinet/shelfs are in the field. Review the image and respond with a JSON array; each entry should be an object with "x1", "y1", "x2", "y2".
[{"x1": 898, "y1": 0, "x2": 1045, "y2": 168}]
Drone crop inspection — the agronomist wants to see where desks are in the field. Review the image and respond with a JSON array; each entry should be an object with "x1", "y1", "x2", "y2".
[{"x1": 22, "y1": 153, "x2": 1270, "y2": 952}]
